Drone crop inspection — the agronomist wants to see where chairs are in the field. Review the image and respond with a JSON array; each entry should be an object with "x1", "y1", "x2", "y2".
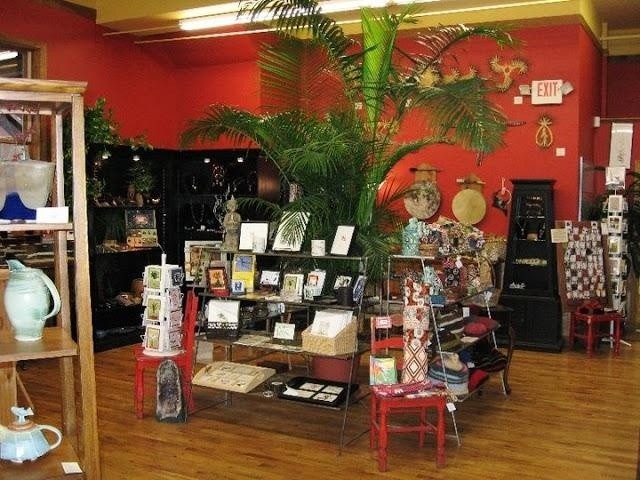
[
  {"x1": 369, "y1": 316, "x2": 446, "y2": 472},
  {"x1": 132, "y1": 291, "x2": 199, "y2": 419}
]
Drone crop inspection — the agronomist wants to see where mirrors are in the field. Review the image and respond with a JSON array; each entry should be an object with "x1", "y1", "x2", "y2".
[{"x1": 0, "y1": 34, "x2": 51, "y2": 162}]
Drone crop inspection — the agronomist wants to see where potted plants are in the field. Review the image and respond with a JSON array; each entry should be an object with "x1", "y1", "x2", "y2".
[{"x1": 178, "y1": 0, "x2": 527, "y2": 387}]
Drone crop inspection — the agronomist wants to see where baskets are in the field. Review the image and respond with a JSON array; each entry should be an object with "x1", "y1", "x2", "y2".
[{"x1": 301, "y1": 315, "x2": 359, "y2": 356}]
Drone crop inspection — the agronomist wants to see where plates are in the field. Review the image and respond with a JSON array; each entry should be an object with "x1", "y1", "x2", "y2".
[{"x1": 108, "y1": 326, "x2": 137, "y2": 335}]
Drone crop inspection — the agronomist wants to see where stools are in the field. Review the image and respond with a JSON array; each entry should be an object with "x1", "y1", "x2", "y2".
[
  {"x1": 569, "y1": 310, "x2": 621, "y2": 358},
  {"x1": 469, "y1": 304, "x2": 515, "y2": 396}
]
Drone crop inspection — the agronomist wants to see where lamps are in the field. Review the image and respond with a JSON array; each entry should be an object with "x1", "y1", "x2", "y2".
[{"x1": 179, "y1": 0, "x2": 440, "y2": 31}]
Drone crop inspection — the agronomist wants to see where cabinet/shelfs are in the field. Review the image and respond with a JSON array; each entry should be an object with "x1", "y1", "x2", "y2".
[
  {"x1": 490, "y1": 179, "x2": 566, "y2": 353},
  {"x1": 184, "y1": 245, "x2": 384, "y2": 457},
  {"x1": 68, "y1": 142, "x2": 177, "y2": 353},
  {"x1": 384, "y1": 255, "x2": 508, "y2": 446},
  {"x1": 179, "y1": 148, "x2": 280, "y2": 333},
  {"x1": 0, "y1": 77, "x2": 103, "y2": 480}
]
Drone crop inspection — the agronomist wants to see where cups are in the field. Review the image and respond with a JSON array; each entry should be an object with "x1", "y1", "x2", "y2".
[{"x1": 418, "y1": 243, "x2": 440, "y2": 256}]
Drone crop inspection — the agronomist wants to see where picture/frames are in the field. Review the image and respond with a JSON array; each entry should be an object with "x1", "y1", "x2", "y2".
[
  {"x1": 184, "y1": 240, "x2": 228, "y2": 282},
  {"x1": 259, "y1": 268, "x2": 280, "y2": 291},
  {"x1": 272, "y1": 210, "x2": 310, "y2": 253},
  {"x1": 329, "y1": 222, "x2": 355, "y2": 256},
  {"x1": 205, "y1": 266, "x2": 229, "y2": 293},
  {"x1": 238, "y1": 221, "x2": 269, "y2": 252}
]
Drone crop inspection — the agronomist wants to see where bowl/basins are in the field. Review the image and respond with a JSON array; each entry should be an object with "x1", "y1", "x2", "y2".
[{"x1": 0, "y1": 161, "x2": 56, "y2": 211}]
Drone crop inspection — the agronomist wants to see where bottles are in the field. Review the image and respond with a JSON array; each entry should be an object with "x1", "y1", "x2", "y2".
[{"x1": 403, "y1": 218, "x2": 419, "y2": 255}]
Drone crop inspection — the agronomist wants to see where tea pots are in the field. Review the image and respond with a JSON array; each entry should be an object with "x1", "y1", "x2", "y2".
[
  {"x1": 0, "y1": 405, "x2": 63, "y2": 465},
  {"x1": 4, "y1": 258, "x2": 63, "y2": 342}
]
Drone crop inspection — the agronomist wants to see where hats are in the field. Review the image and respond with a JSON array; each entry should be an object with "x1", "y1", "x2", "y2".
[
  {"x1": 474, "y1": 338, "x2": 507, "y2": 372},
  {"x1": 436, "y1": 309, "x2": 465, "y2": 333},
  {"x1": 433, "y1": 331, "x2": 465, "y2": 352},
  {"x1": 430, "y1": 353, "x2": 471, "y2": 395}
]
